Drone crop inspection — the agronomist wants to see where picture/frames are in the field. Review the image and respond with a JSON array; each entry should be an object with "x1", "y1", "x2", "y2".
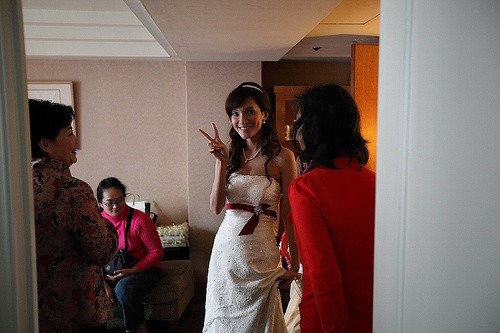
[{"x1": 28, "y1": 79, "x2": 79, "y2": 150}]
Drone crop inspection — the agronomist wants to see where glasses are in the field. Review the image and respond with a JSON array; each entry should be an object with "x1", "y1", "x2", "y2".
[{"x1": 99, "y1": 198, "x2": 124, "y2": 206}]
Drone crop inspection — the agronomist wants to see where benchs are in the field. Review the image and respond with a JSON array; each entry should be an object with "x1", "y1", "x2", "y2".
[{"x1": 112, "y1": 260, "x2": 195, "y2": 333}]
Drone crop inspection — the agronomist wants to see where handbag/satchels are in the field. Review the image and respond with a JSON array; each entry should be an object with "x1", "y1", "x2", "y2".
[
  {"x1": 101, "y1": 207, "x2": 139, "y2": 278},
  {"x1": 124, "y1": 192, "x2": 151, "y2": 217}
]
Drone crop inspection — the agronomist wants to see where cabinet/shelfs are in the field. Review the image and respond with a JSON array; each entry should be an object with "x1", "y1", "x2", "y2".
[{"x1": 273, "y1": 85, "x2": 350, "y2": 240}]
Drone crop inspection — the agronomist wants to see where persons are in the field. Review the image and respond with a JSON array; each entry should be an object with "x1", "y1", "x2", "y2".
[
  {"x1": 29, "y1": 99, "x2": 119, "y2": 333},
  {"x1": 199, "y1": 81, "x2": 300, "y2": 333},
  {"x1": 97, "y1": 177, "x2": 168, "y2": 333},
  {"x1": 280, "y1": 83, "x2": 376, "y2": 333}
]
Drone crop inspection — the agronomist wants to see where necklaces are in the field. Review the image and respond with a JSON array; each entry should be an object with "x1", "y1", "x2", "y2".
[{"x1": 242, "y1": 145, "x2": 262, "y2": 164}]
